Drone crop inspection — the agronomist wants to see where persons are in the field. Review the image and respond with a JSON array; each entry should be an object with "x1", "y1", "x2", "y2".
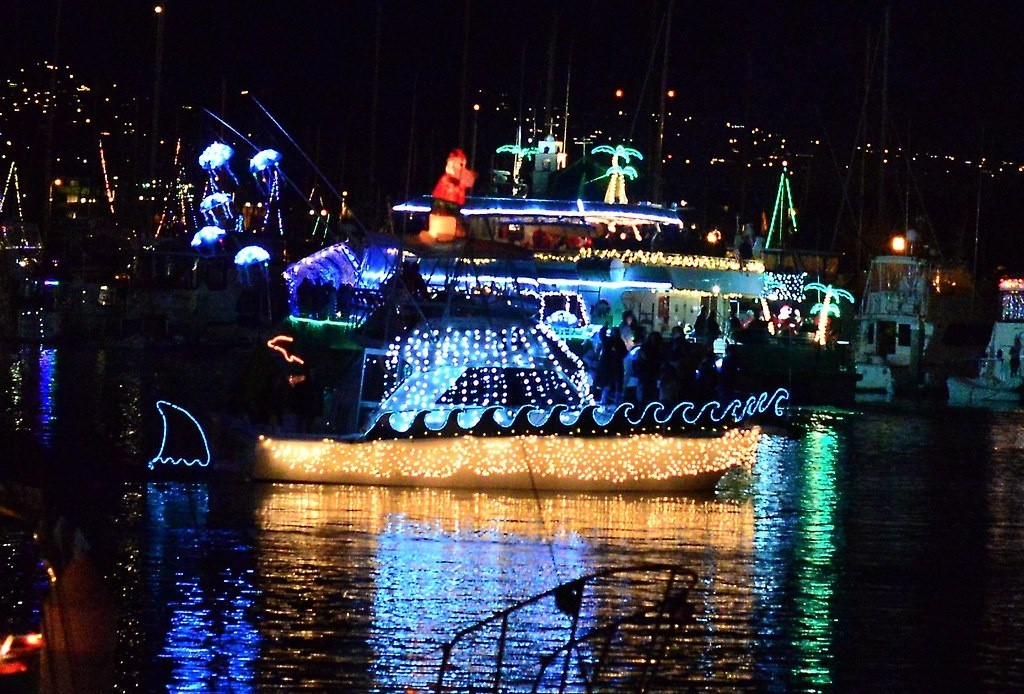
[
  {"x1": 1009, "y1": 337, "x2": 1022, "y2": 378},
  {"x1": 279, "y1": 265, "x2": 830, "y2": 444}
]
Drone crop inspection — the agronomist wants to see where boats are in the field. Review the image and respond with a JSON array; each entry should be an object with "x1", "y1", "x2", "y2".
[{"x1": 9, "y1": 12, "x2": 1018, "y2": 480}]
